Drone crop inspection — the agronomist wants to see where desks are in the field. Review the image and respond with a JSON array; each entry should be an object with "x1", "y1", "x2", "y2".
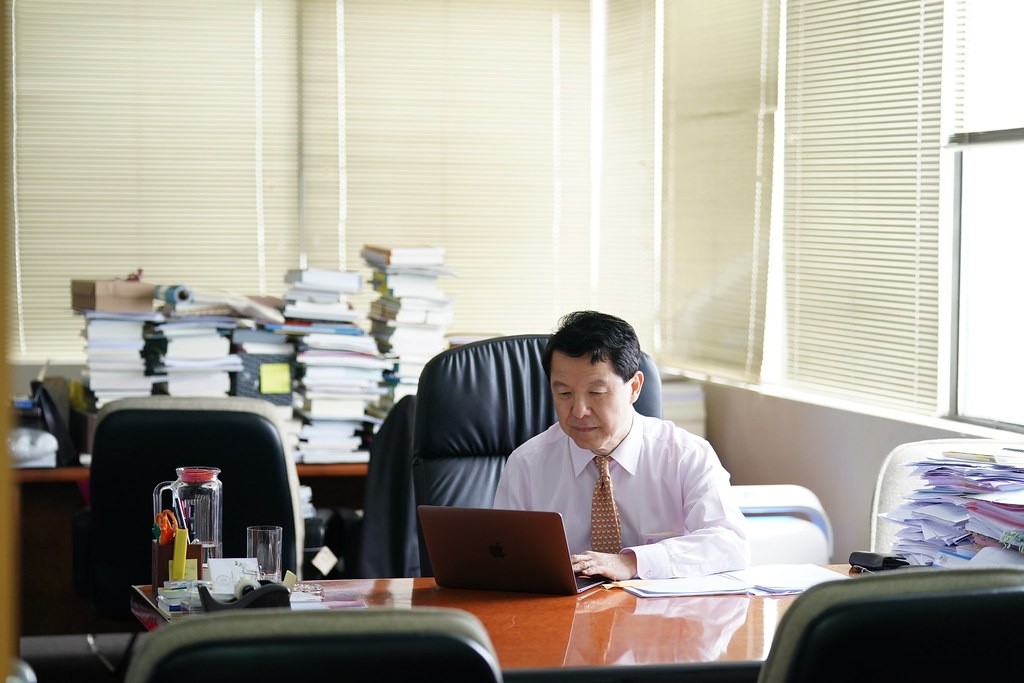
[
  {"x1": 7, "y1": 463, "x2": 368, "y2": 635},
  {"x1": 129, "y1": 564, "x2": 871, "y2": 683}
]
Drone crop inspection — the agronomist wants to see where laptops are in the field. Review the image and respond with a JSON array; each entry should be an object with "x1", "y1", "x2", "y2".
[{"x1": 417, "y1": 506, "x2": 609, "y2": 594}]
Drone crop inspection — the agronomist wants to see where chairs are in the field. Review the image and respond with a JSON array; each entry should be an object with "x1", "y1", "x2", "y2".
[
  {"x1": 412, "y1": 334, "x2": 663, "y2": 579},
  {"x1": 88, "y1": 396, "x2": 304, "y2": 580},
  {"x1": 84, "y1": 409, "x2": 297, "y2": 677},
  {"x1": 757, "y1": 566, "x2": 1024, "y2": 683},
  {"x1": 125, "y1": 608, "x2": 504, "y2": 683}
]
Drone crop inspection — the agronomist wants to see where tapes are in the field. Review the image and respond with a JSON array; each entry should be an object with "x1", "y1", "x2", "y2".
[{"x1": 234, "y1": 576, "x2": 261, "y2": 601}]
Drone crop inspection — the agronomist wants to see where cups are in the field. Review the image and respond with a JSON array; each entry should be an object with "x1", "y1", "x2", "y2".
[{"x1": 248, "y1": 526, "x2": 282, "y2": 584}]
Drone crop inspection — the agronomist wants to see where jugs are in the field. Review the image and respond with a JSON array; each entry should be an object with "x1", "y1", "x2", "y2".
[{"x1": 153, "y1": 466, "x2": 223, "y2": 577}]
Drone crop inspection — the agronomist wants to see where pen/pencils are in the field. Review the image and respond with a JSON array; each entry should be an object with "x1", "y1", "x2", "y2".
[{"x1": 152, "y1": 497, "x2": 197, "y2": 544}]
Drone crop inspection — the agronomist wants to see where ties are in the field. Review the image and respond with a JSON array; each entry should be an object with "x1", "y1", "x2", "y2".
[{"x1": 590, "y1": 455, "x2": 623, "y2": 555}]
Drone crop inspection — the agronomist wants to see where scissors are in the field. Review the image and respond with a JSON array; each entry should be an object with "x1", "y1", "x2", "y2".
[{"x1": 156, "y1": 509, "x2": 178, "y2": 533}]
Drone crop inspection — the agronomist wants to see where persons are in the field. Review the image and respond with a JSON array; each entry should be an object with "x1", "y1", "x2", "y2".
[{"x1": 493, "y1": 311, "x2": 750, "y2": 581}]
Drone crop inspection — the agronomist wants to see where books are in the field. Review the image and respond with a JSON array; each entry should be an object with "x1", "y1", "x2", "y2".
[
  {"x1": 157, "y1": 581, "x2": 211, "y2": 616},
  {"x1": 71, "y1": 243, "x2": 505, "y2": 464}
]
